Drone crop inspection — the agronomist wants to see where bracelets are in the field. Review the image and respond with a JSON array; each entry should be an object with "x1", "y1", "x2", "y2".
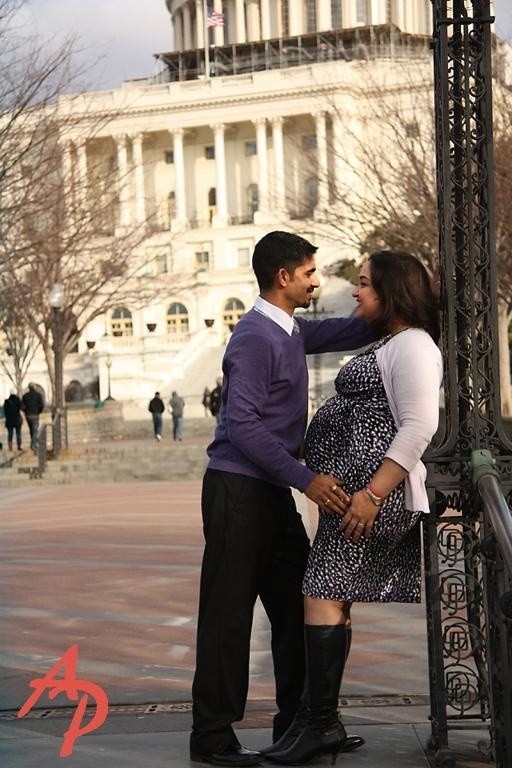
[{"x1": 366, "y1": 483, "x2": 385, "y2": 500}]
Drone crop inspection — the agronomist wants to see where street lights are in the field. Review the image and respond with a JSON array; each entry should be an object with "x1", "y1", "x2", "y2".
[
  {"x1": 309, "y1": 283, "x2": 324, "y2": 402},
  {"x1": 43, "y1": 280, "x2": 68, "y2": 453},
  {"x1": 104, "y1": 351, "x2": 115, "y2": 401}
]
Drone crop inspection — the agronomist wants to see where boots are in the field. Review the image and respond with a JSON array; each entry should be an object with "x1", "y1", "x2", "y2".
[{"x1": 260, "y1": 623, "x2": 365, "y2": 766}]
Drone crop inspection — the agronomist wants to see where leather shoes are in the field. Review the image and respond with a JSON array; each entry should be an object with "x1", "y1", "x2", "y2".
[{"x1": 190, "y1": 741, "x2": 264, "y2": 765}]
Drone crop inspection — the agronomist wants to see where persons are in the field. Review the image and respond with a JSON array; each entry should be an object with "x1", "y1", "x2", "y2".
[
  {"x1": 23, "y1": 383, "x2": 43, "y2": 451},
  {"x1": 148, "y1": 391, "x2": 165, "y2": 441},
  {"x1": 190, "y1": 225, "x2": 374, "y2": 768},
  {"x1": 167, "y1": 390, "x2": 186, "y2": 441},
  {"x1": 208, "y1": 376, "x2": 223, "y2": 425},
  {"x1": 258, "y1": 250, "x2": 445, "y2": 766},
  {"x1": 201, "y1": 386, "x2": 213, "y2": 416},
  {"x1": 3, "y1": 387, "x2": 27, "y2": 450}
]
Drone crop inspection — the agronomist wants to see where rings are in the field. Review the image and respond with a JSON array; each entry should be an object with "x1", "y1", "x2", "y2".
[
  {"x1": 324, "y1": 498, "x2": 330, "y2": 506},
  {"x1": 331, "y1": 484, "x2": 338, "y2": 491}
]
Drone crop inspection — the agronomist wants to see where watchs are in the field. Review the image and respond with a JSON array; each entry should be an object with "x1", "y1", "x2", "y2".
[{"x1": 364, "y1": 487, "x2": 383, "y2": 506}]
